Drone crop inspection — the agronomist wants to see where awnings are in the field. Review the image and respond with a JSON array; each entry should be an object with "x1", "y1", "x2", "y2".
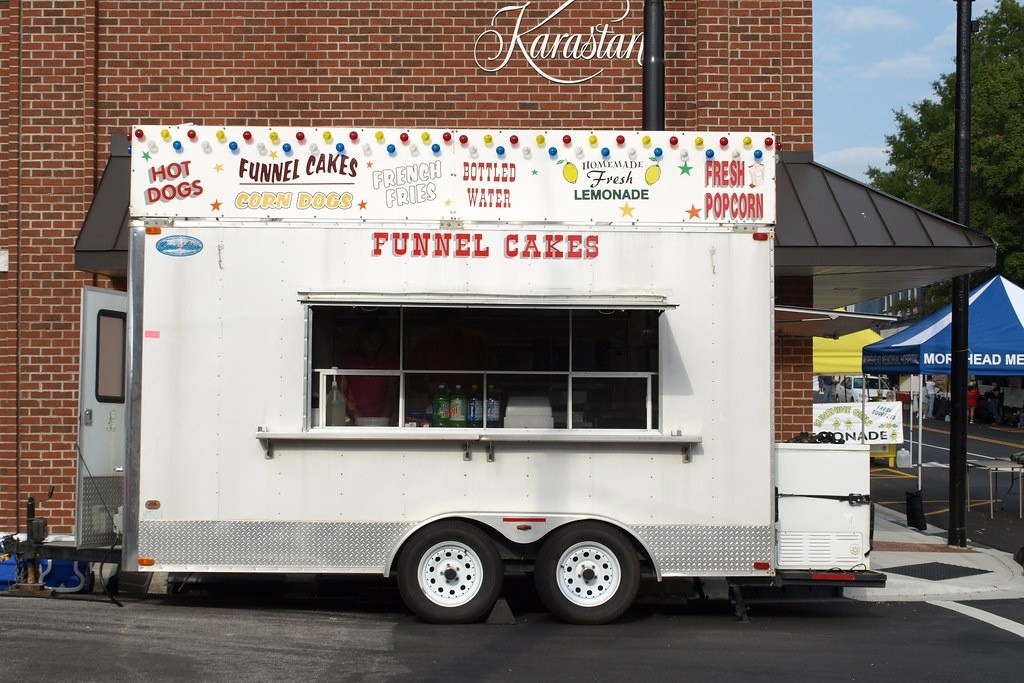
[
  {"x1": 70, "y1": 133, "x2": 130, "y2": 277},
  {"x1": 775, "y1": 154, "x2": 999, "y2": 314}
]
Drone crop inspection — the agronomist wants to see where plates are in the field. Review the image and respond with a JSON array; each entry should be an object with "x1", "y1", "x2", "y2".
[{"x1": 354, "y1": 416, "x2": 390, "y2": 426}]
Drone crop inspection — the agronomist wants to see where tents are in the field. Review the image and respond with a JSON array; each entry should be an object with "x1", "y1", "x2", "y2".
[
  {"x1": 860, "y1": 274, "x2": 1024, "y2": 491},
  {"x1": 813, "y1": 334, "x2": 870, "y2": 402},
  {"x1": 836, "y1": 327, "x2": 889, "y2": 398}
]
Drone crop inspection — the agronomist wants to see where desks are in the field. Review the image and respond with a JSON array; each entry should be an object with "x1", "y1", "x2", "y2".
[{"x1": 967, "y1": 460, "x2": 1024, "y2": 519}]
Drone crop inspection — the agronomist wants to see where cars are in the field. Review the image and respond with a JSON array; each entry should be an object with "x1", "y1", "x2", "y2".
[{"x1": 835, "y1": 377, "x2": 894, "y2": 404}]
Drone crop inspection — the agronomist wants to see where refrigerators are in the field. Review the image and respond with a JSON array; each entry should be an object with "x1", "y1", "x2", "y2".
[{"x1": 775, "y1": 443, "x2": 871, "y2": 571}]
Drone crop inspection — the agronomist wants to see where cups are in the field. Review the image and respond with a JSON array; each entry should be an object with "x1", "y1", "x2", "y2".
[
  {"x1": 407, "y1": 411, "x2": 424, "y2": 419},
  {"x1": 426, "y1": 412, "x2": 432, "y2": 422}
]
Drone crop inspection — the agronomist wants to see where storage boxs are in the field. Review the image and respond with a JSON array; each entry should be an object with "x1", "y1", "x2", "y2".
[
  {"x1": 504, "y1": 416, "x2": 555, "y2": 427},
  {"x1": 505, "y1": 395, "x2": 553, "y2": 417}
]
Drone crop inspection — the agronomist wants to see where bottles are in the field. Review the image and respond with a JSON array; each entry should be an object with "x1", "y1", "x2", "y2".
[
  {"x1": 487, "y1": 384, "x2": 502, "y2": 427},
  {"x1": 432, "y1": 384, "x2": 449, "y2": 427},
  {"x1": 896, "y1": 447, "x2": 910, "y2": 468},
  {"x1": 467, "y1": 384, "x2": 482, "y2": 427},
  {"x1": 449, "y1": 384, "x2": 467, "y2": 427},
  {"x1": 345, "y1": 416, "x2": 352, "y2": 425}
]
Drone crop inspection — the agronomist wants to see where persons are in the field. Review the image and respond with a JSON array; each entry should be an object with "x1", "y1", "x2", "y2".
[
  {"x1": 817, "y1": 376, "x2": 835, "y2": 403},
  {"x1": 987, "y1": 379, "x2": 1002, "y2": 427},
  {"x1": 924, "y1": 373, "x2": 936, "y2": 419},
  {"x1": 334, "y1": 321, "x2": 394, "y2": 418},
  {"x1": 967, "y1": 381, "x2": 979, "y2": 425}
]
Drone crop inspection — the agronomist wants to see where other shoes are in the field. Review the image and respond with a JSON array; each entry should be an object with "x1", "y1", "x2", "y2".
[
  {"x1": 821, "y1": 392, "x2": 824, "y2": 394},
  {"x1": 970, "y1": 420, "x2": 974, "y2": 424},
  {"x1": 923, "y1": 415, "x2": 928, "y2": 419},
  {"x1": 819, "y1": 392, "x2": 821, "y2": 394},
  {"x1": 916, "y1": 415, "x2": 919, "y2": 418}
]
